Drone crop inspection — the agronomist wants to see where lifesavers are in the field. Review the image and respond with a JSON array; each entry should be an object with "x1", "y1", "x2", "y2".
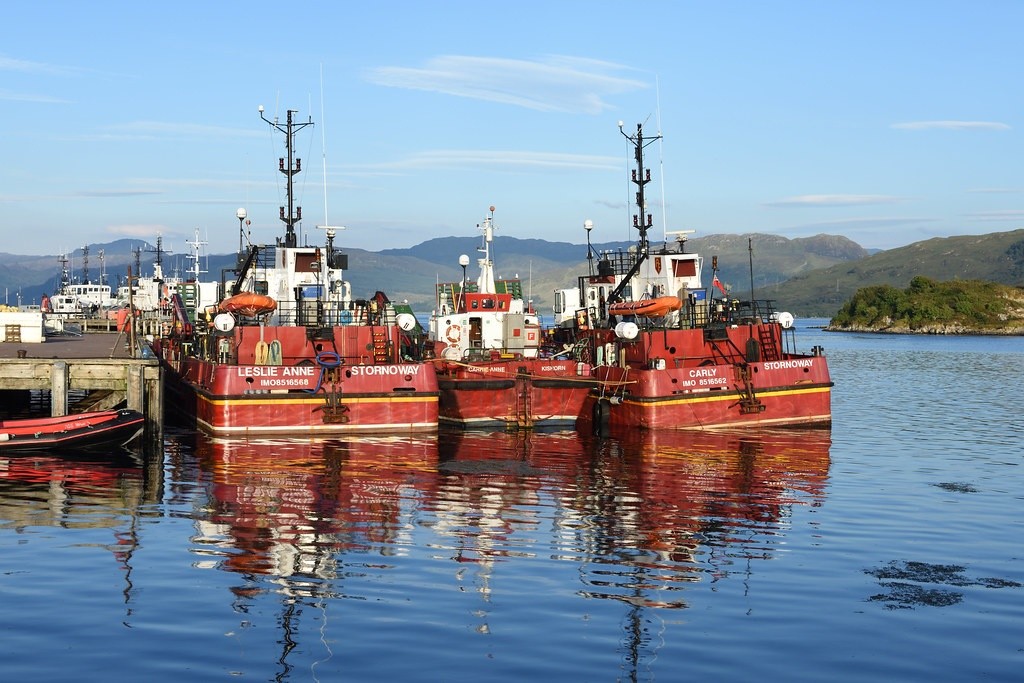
[
  {"x1": 445, "y1": 324, "x2": 462, "y2": 342},
  {"x1": 340, "y1": 309, "x2": 352, "y2": 324}
]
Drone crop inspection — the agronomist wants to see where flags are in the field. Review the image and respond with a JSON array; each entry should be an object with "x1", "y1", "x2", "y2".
[{"x1": 712, "y1": 270, "x2": 727, "y2": 296}]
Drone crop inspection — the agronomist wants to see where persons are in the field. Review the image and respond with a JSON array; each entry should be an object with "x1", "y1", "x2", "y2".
[
  {"x1": 606, "y1": 290, "x2": 618, "y2": 331},
  {"x1": 339, "y1": 298, "x2": 378, "y2": 325},
  {"x1": 218, "y1": 336, "x2": 235, "y2": 365}
]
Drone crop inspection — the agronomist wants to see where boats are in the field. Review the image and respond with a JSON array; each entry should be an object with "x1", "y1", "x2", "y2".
[
  {"x1": 542, "y1": 119, "x2": 834, "y2": 433},
  {"x1": 49, "y1": 227, "x2": 221, "y2": 356},
  {"x1": 0, "y1": 409, "x2": 146, "y2": 452},
  {"x1": 428, "y1": 205, "x2": 602, "y2": 429},
  {"x1": 150, "y1": 103, "x2": 440, "y2": 434}
]
